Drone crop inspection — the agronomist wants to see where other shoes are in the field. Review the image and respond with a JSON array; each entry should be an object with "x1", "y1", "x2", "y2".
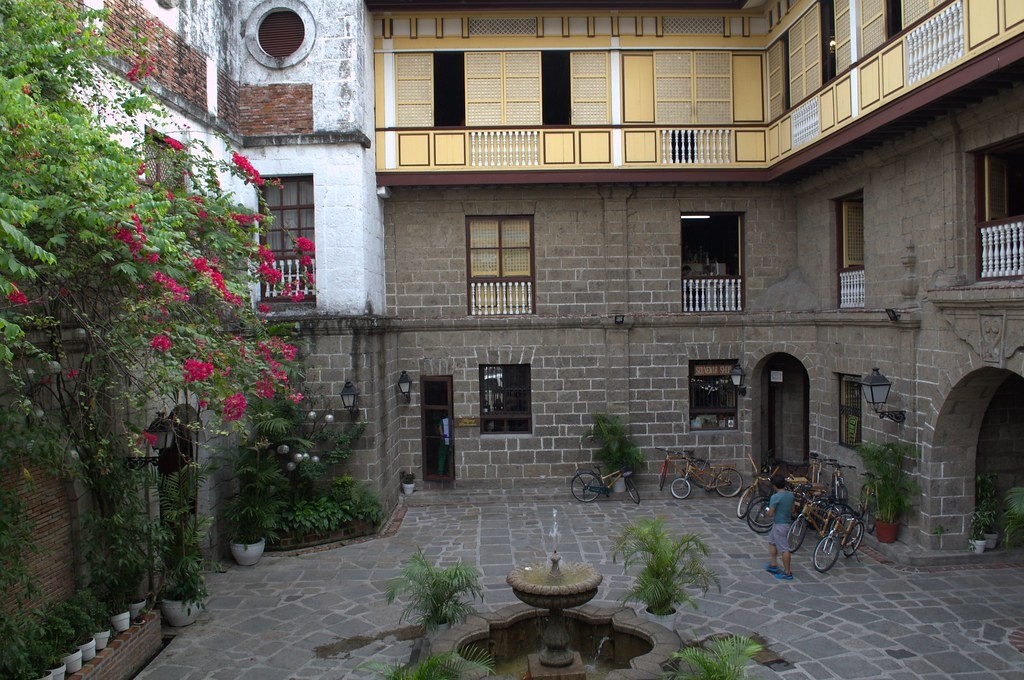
[
  {"x1": 775, "y1": 570, "x2": 793, "y2": 580},
  {"x1": 764, "y1": 563, "x2": 779, "y2": 573}
]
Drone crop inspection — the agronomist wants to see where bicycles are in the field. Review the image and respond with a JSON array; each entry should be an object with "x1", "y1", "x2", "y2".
[
  {"x1": 654, "y1": 445, "x2": 877, "y2": 574},
  {"x1": 570, "y1": 461, "x2": 640, "y2": 504}
]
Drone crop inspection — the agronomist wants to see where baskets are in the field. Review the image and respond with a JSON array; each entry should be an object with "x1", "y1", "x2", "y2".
[{"x1": 619, "y1": 464, "x2": 633, "y2": 478}]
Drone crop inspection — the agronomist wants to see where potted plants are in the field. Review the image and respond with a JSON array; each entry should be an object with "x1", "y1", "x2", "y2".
[
  {"x1": 17, "y1": 558, "x2": 149, "y2": 680},
  {"x1": 400, "y1": 471, "x2": 415, "y2": 494},
  {"x1": 217, "y1": 418, "x2": 316, "y2": 567},
  {"x1": 579, "y1": 411, "x2": 647, "y2": 492},
  {"x1": 140, "y1": 456, "x2": 224, "y2": 627},
  {"x1": 968, "y1": 471, "x2": 1003, "y2": 553},
  {"x1": 612, "y1": 517, "x2": 722, "y2": 632},
  {"x1": 855, "y1": 438, "x2": 919, "y2": 544},
  {"x1": 384, "y1": 544, "x2": 485, "y2": 634}
]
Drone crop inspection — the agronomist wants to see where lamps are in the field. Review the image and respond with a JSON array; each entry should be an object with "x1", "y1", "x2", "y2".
[
  {"x1": 398, "y1": 369, "x2": 412, "y2": 403},
  {"x1": 859, "y1": 367, "x2": 906, "y2": 424},
  {"x1": 731, "y1": 363, "x2": 746, "y2": 397},
  {"x1": 886, "y1": 308, "x2": 901, "y2": 321},
  {"x1": 615, "y1": 315, "x2": 625, "y2": 323},
  {"x1": 122, "y1": 411, "x2": 179, "y2": 473},
  {"x1": 340, "y1": 379, "x2": 359, "y2": 418}
]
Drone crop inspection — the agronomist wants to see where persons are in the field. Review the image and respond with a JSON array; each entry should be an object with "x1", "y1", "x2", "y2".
[
  {"x1": 764, "y1": 474, "x2": 796, "y2": 580},
  {"x1": 436, "y1": 410, "x2": 451, "y2": 475}
]
